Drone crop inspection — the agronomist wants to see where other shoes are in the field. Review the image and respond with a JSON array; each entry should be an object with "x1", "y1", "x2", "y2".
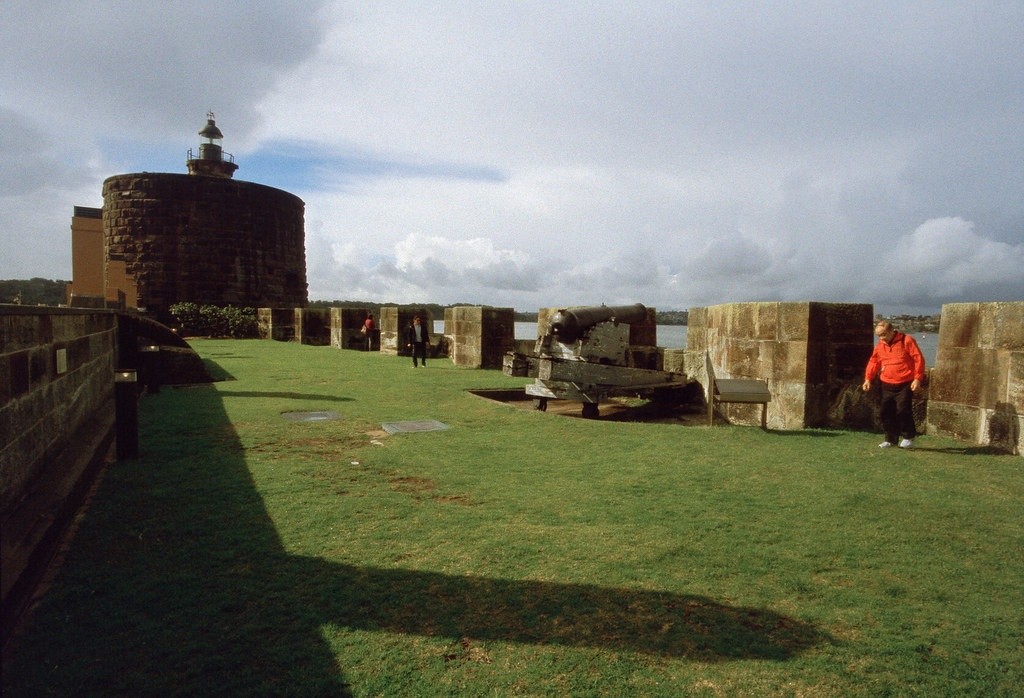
[
  {"x1": 422, "y1": 365, "x2": 425, "y2": 367},
  {"x1": 412, "y1": 365, "x2": 417, "y2": 368}
]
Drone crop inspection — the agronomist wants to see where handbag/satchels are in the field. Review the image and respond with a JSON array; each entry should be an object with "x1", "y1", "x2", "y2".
[{"x1": 361, "y1": 325, "x2": 367, "y2": 334}]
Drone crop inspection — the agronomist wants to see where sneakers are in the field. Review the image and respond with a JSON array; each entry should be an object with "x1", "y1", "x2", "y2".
[
  {"x1": 900, "y1": 438, "x2": 911, "y2": 448},
  {"x1": 878, "y1": 441, "x2": 892, "y2": 447}
]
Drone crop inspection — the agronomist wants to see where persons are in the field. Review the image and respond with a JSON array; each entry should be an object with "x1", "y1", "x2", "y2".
[
  {"x1": 407, "y1": 314, "x2": 430, "y2": 368},
  {"x1": 365, "y1": 313, "x2": 377, "y2": 351},
  {"x1": 861, "y1": 319, "x2": 926, "y2": 450}
]
